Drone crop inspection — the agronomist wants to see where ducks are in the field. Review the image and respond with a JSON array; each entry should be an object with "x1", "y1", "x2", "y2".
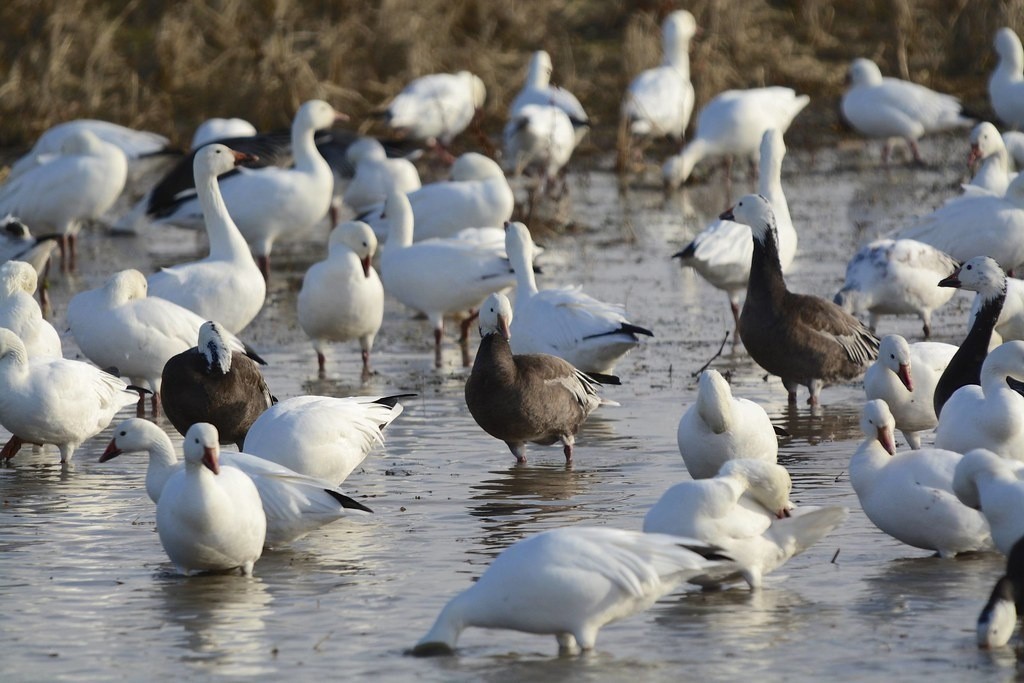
[
  {"x1": 465, "y1": 293, "x2": 622, "y2": 468},
  {"x1": 0, "y1": 49, "x2": 597, "y2": 578},
  {"x1": 502, "y1": 222, "x2": 655, "y2": 373},
  {"x1": 643, "y1": 459, "x2": 851, "y2": 592},
  {"x1": 832, "y1": 24, "x2": 1023, "y2": 655},
  {"x1": 670, "y1": 128, "x2": 797, "y2": 330},
  {"x1": 676, "y1": 369, "x2": 789, "y2": 479},
  {"x1": 621, "y1": 9, "x2": 707, "y2": 187},
  {"x1": 718, "y1": 193, "x2": 880, "y2": 411},
  {"x1": 658, "y1": 84, "x2": 811, "y2": 200},
  {"x1": 403, "y1": 525, "x2": 738, "y2": 659}
]
